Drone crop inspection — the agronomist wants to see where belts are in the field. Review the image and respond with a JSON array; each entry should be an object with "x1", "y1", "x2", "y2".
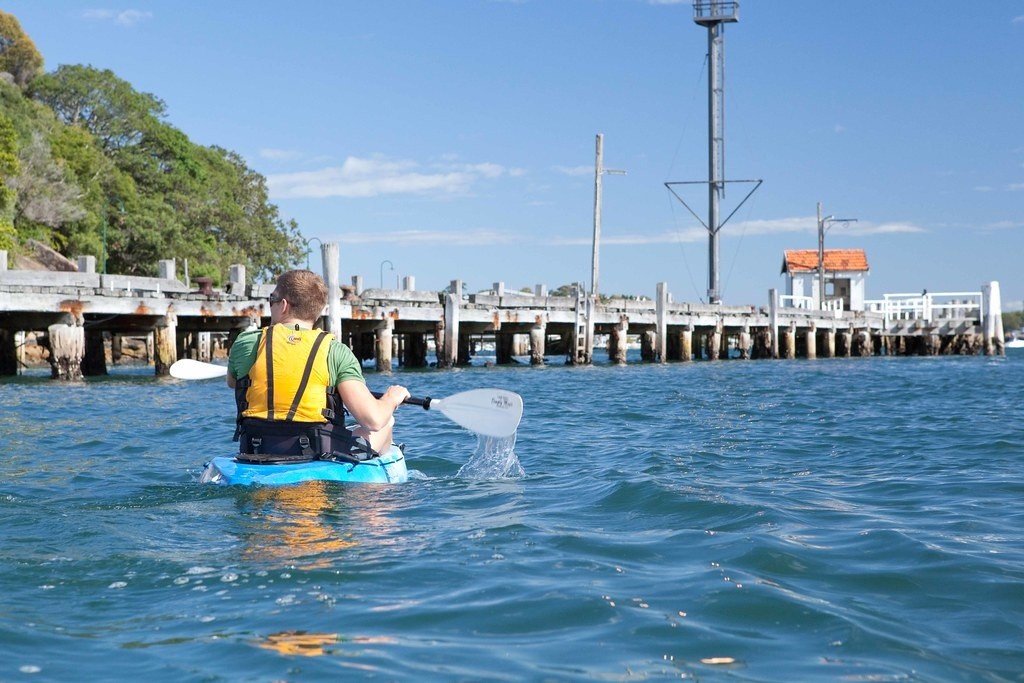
[{"x1": 243, "y1": 426, "x2": 316, "y2": 436}]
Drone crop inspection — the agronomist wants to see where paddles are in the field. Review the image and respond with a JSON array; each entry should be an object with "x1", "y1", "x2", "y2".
[{"x1": 169, "y1": 358, "x2": 524, "y2": 436}]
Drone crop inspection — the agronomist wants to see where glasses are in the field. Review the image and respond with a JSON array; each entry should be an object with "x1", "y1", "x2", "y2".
[{"x1": 268, "y1": 293, "x2": 298, "y2": 308}]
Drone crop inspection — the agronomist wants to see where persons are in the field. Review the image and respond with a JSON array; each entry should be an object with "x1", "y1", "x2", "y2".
[{"x1": 226, "y1": 269, "x2": 410, "y2": 470}]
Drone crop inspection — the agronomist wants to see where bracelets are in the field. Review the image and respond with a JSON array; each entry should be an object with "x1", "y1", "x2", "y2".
[{"x1": 382, "y1": 394, "x2": 398, "y2": 411}]
